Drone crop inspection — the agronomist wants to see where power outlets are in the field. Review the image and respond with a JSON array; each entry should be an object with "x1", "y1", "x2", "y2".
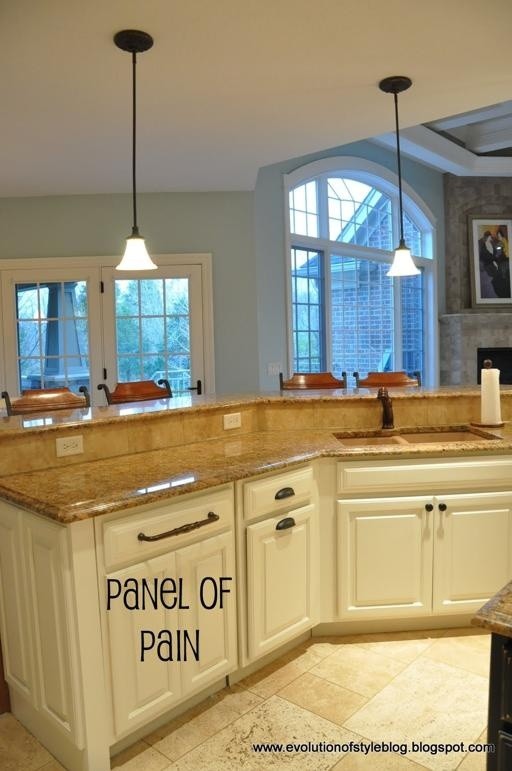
[
  {"x1": 55, "y1": 435, "x2": 85, "y2": 458},
  {"x1": 223, "y1": 412, "x2": 243, "y2": 431}
]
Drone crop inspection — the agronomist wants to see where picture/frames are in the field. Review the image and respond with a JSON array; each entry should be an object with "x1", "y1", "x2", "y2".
[{"x1": 467, "y1": 213, "x2": 512, "y2": 308}]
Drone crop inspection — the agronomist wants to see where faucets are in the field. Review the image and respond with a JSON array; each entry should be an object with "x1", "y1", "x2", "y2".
[{"x1": 376, "y1": 386, "x2": 396, "y2": 429}]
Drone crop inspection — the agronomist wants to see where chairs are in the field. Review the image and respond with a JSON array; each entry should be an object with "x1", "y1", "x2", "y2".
[
  {"x1": 353, "y1": 371, "x2": 421, "y2": 389},
  {"x1": 98, "y1": 379, "x2": 173, "y2": 406},
  {"x1": 278, "y1": 372, "x2": 347, "y2": 388},
  {"x1": 2, "y1": 385, "x2": 90, "y2": 417}
]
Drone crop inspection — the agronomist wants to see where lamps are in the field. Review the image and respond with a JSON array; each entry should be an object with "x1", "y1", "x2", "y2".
[
  {"x1": 111, "y1": 29, "x2": 160, "y2": 272},
  {"x1": 378, "y1": 76, "x2": 423, "y2": 277}
]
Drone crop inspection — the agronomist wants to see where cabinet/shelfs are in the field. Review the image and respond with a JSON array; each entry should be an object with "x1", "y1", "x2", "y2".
[
  {"x1": 323, "y1": 450, "x2": 512, "y2": 638},
  {"x1": 486, "y1": 634, "x2": 512, "y2": 771},
  {"x1": 1, "y1": 457, "x2": 323, "y2": 770}
]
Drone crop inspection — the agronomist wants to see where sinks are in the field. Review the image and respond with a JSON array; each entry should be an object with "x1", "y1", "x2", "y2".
[
  {"x1": 397, "y1": 420, "x2": 503, "y2": 446},
  {"x1": 329, "y1": 423, "x2": 404, "y2": 449}
]
